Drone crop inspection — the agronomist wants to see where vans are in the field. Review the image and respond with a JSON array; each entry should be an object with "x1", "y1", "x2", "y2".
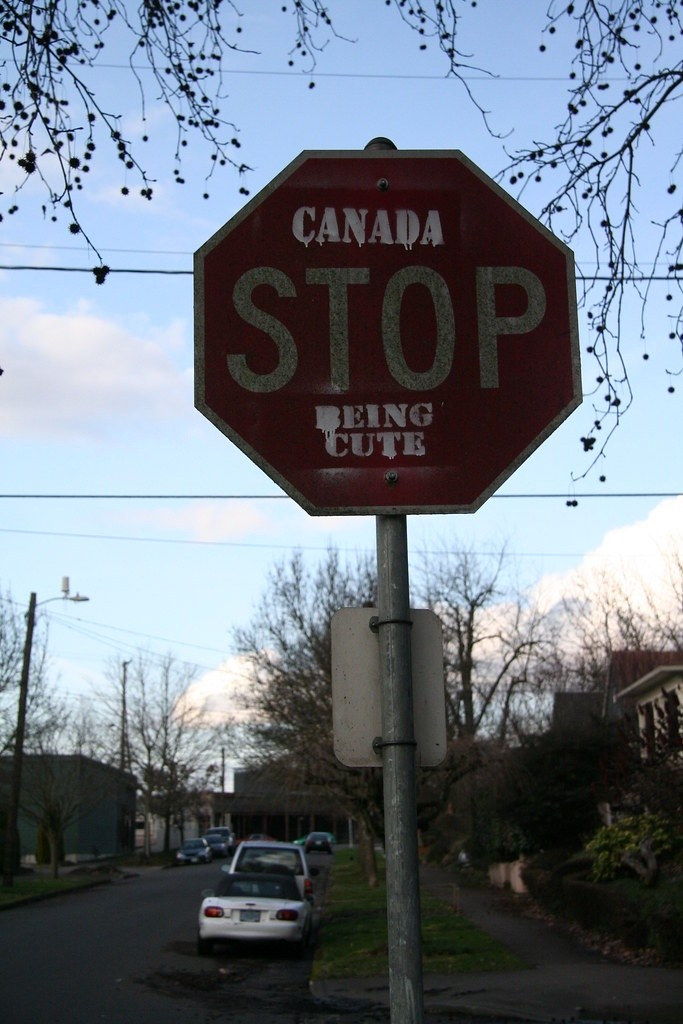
[{"x1": 225, "y1": 841, "x2": 313, "y2": 906}]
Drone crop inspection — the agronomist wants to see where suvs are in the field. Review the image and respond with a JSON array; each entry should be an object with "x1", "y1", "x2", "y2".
[
  {"x1": 305, "y1": 832, "x2": 333, "y2": 855},
  {"x1": 205, "y1": 827, "x2": 235, "y2": 856}
]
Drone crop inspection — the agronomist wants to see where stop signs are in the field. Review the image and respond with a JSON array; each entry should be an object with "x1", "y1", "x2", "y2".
[{"x1": 195, "y1": 149, "x2": 582, "y2": 515}]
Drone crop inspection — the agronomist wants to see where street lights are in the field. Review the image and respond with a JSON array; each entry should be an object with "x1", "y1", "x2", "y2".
[{"x1": 4, "y1": 573, "x2": 89, "y2": 874}]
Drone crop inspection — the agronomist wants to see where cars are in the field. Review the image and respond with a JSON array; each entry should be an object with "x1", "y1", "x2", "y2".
[
  {"x1": 198, "y1": 871, "x2": 312, "y2": 952},
  {"x1": 202, "y1": 834, "x2": 225, "y2": 854},
  {"x1": 175, "y1": 839, "x2": 211, "y2": 864}
]
